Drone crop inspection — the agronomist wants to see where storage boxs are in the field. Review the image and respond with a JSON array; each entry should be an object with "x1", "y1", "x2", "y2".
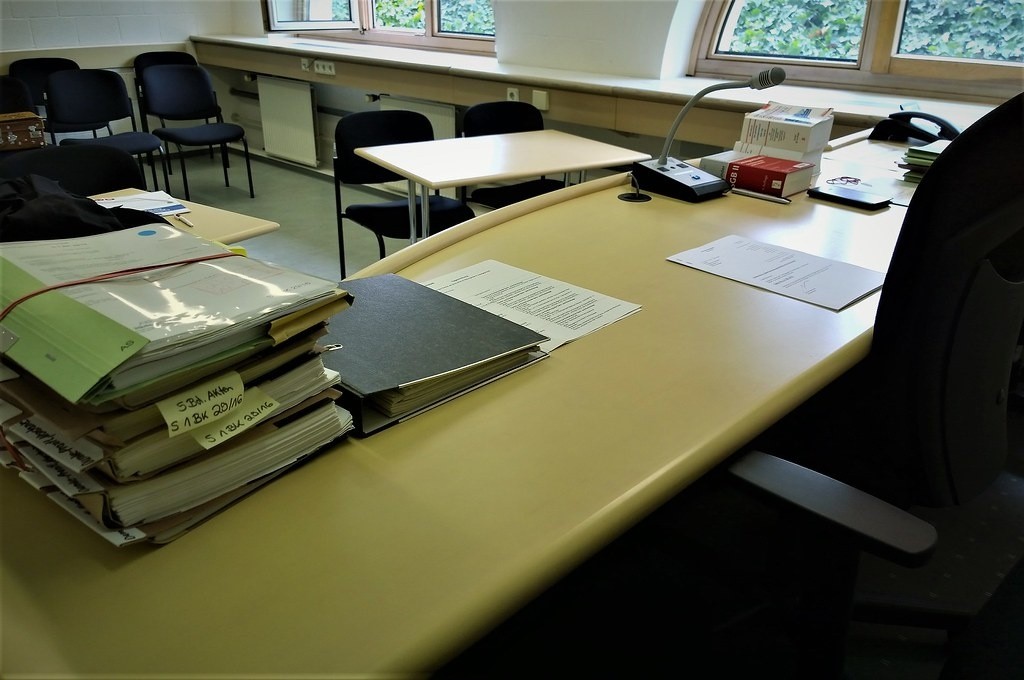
[{"x1": 0, "y1": 112, "x2": 46, "y2": 150}]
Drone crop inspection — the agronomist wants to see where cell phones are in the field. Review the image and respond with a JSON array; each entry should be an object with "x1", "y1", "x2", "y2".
[{"x1": 806, "y1": 185, "x2": 891, "y2": 211}]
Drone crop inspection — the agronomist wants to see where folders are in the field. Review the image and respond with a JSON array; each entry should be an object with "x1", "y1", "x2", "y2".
[
  {"x1": 0, "y1": 220, "x2": 354, "y2": 552},
  {"x1": 310, "y1": 272, "x2": 550, "y2": 439}
]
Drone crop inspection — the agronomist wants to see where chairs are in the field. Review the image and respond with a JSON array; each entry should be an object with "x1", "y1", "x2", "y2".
[
  {"x1": 132, "y1": 49, "x2": 256, "y2": 199},
  {"x1": 0, "y1": 58, "x2": 172, "y2": 197},
  {"x1": 685, "y1": 91, "x2": 1024, "y2": 680}
]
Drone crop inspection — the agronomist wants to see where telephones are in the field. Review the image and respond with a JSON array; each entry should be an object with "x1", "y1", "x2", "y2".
[{"x1": 869, "y1": 108, "x2": 961, "y2": 145}]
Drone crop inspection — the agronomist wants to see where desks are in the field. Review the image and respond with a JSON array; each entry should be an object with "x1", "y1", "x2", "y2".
[
  {"x1": 353, "y1": 129, "x2": 653, "y2": 244},
  {"x1": 87, "y1": 188, "x2": 281, "y2": 245},
  {"x1": 189, "y1": 35, "x2": 997, "y2": 168}
]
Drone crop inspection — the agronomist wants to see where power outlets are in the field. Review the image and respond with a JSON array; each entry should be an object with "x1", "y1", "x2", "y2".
[{"x1": 314, "y1": 60, "x2": 336, "y2": 75}]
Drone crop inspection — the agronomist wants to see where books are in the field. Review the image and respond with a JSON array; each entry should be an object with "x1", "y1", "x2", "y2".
[
  {"x1": 899, "y1": 138, "x2": 960, "y2": 185},
  {"x1": 699, "y1": 101, "x2": 836, "y2": 201}
]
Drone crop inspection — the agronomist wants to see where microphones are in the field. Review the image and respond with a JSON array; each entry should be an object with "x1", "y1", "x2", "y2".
[{"x1": 633, "y1": 66, "x2": 786, "y2": 203}]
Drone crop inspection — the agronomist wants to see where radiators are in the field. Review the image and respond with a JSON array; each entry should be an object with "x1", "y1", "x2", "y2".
[
  {"x1": 257, "y1": 75, "x2": 322, "y2": 168},
  {"x1": 378, "y1": 94, "x2": 458, "y2": 200}
]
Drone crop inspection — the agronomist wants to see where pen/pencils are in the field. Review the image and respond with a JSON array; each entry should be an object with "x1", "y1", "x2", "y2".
[
  {"x1": 174, "y1": 213, "x2": 194, "y2": 228},
  {"x1": 731, "y1": 187, "x2": 791, "y2": 204}
]
